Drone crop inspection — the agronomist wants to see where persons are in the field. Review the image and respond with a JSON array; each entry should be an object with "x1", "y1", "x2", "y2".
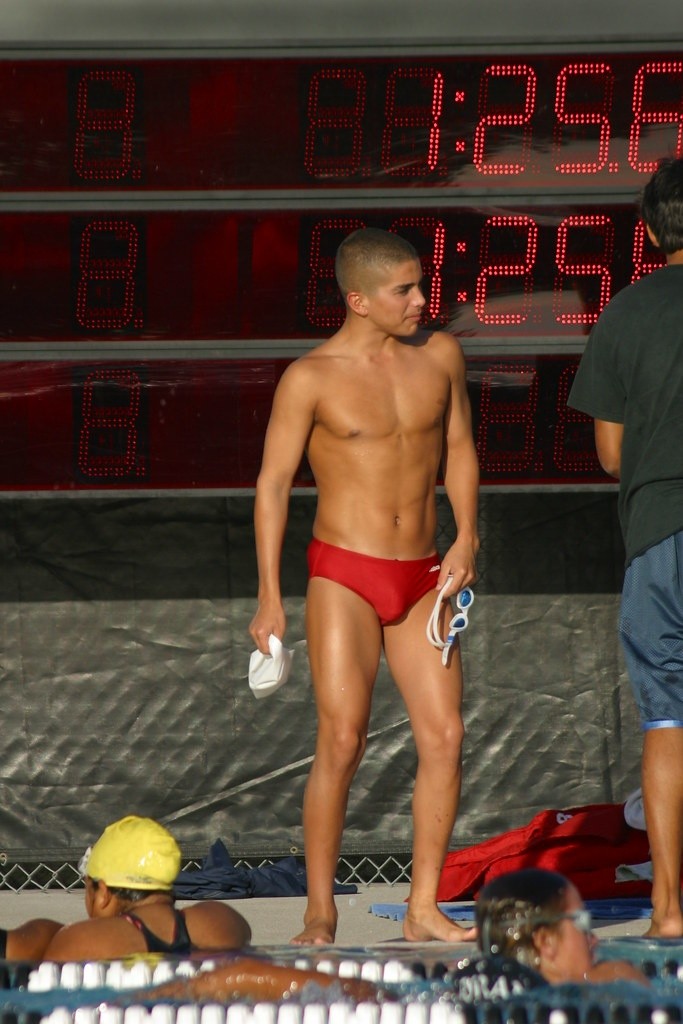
[
  {"x1": 565, "y1": 157, "x2": 683, "y2": 941},
  {"x1": 475, "y1": 870, "x2": 651, "y2": 987},
  {"x1": 138, "y1": 864, "x2": 650, "y2": 1001},
  {"x1": 40, "y1": 814, "x2": 251, "y2": 961},
  {"x1": 250, "y1": 224, "x2": 483, "y2": 946},
  {"x1": 0, "y1": 917, "x2": 64, "y2": 961}
]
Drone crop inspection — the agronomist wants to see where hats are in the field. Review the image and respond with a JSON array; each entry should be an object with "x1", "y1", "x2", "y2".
[{"x1": 81, "y1": 815, "x2": 182, "y2": 890}]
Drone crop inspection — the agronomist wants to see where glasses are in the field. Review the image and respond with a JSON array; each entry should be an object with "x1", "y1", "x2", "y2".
[
  {"x1": 571, "y1": 911, "x2": 595, "y2": 935},
  {"x1": 448, "y1": 586, "x2": 474, "y2": 641}
]
